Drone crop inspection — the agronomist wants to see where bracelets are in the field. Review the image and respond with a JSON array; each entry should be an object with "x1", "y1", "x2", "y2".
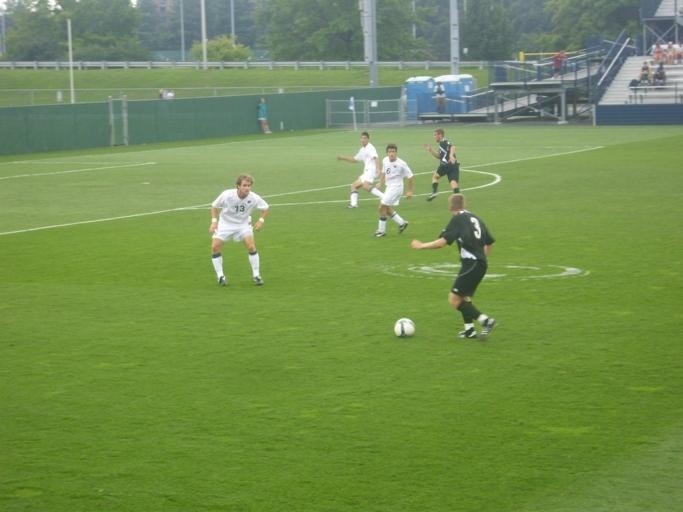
[
  {"x1": 258, "y1": 217, "x2": 265, "y2": 223},
  {"x1": 210, "y1": 217, "x2": 217, "y2": 223}
]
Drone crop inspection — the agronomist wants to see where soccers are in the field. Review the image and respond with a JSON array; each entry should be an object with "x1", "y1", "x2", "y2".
[{"x1": 394, "y1": 318, "x2": 416, "y2": 337}]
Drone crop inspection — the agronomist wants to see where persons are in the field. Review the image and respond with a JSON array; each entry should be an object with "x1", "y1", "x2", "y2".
[
  {"x1": 423, "y1": 128, "x2": 460, "y2": 201},
  {"x1": 434, "y1": 82, "x2": 446, "y2": 113},
  {"x1": 410, "y1": 194, "x2": 497, "y2": 339},
  {"x1": 641, "y1": 41, "x2": 683, "y2": 85},
  {"x1": 551, "y1": 49, "x2": 567, "y2": 80},
  {"x1": 209, "y1": 174, "x2": 270, "y2": 284},
  {"x1": 337, "y1": 131, "x2": 385, "y2": 209},
  {"x1": 258, "y1": 97, "x2": 271, "y2": 134},
  {"x1": 373, "y1": 144, "x2": 413, "y2": 238}
]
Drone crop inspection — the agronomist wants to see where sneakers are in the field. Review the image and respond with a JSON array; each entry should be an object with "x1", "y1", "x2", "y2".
[
  {"x1": 374, "y1": 232, "x2": 385, "y2": 238},
  {"x1": 457, "y1": 328, "x2": 476, "y2": 339},
  {"x1": 218, "y1": 274, "x2": 225, "y2": 286},
  {"x1": 479, "y1": 318, "x2": 496, "y2": 337},
  {"x1": 253, "y1": 277, "x2": 263, "y2": 286},
  {"x1": 397, "y1": 222, "x2": 407, "y2": 236},
  {"x1": 426, "y1": 194, "x2": 436, "y2": 202},
  {"x1": 346, "y1": 205, "x2": 358, "y2": 210}
]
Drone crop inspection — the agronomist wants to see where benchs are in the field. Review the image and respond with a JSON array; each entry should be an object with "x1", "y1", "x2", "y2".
[
  {"x1": 624, "y1": 58, "x2": 683, "y2": 104},
  {"x1": 639, "y1": 1, "x2": 683, "y2": 18}
]
